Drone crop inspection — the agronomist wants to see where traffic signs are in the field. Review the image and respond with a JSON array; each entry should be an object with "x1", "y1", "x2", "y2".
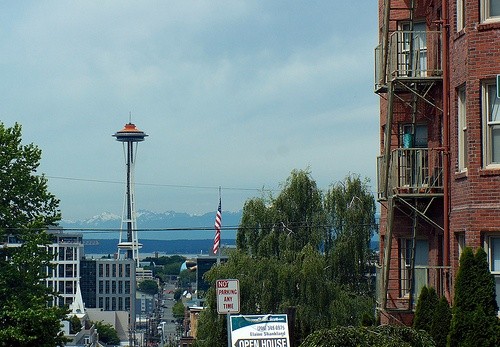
[
  {"x1": 226, "y1": 314, "x2": 290, "y2": 347},
  {"x1": 215, "y1": 279, "x2": 241, "y2": 314}
]
